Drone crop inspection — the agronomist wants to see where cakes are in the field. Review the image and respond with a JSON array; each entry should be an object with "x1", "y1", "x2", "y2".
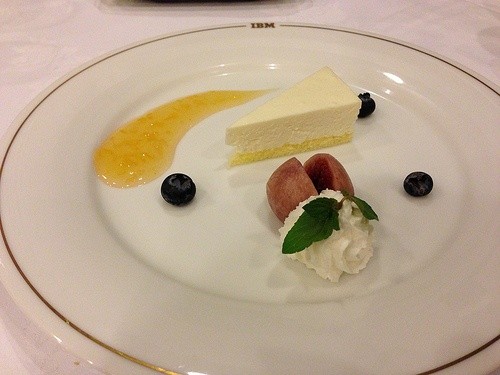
[{"x1": 224, "y1": 67, "x2": 362, "y2": 168}]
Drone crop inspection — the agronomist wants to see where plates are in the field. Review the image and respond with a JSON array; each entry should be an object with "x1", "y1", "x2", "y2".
[{"x1": 0, "y1": 22, "x2": 499, "y2": 375}]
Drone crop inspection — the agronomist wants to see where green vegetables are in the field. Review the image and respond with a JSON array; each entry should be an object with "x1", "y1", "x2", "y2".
[{"x1": 281, "y1": 191, "x2": 379, "y2": 254}]
